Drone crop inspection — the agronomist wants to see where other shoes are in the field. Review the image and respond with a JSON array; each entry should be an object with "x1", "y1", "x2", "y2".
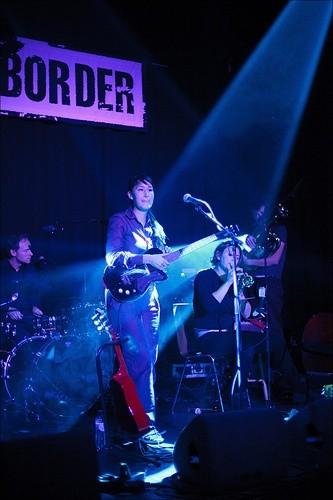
[{"x1": 139, "y1": 429, "x2": 164, "y2": 445}]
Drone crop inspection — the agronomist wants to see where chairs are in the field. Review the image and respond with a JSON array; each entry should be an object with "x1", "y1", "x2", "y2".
[
  {"x1": 169, "y1": 301, "x2": 226, "y2": 415},
  {"x1": 301, "y1": 313, "x2": 333, "y2": 404}
]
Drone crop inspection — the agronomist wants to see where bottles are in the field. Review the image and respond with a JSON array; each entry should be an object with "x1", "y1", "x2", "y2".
[{"x1": 94, "y1": 407, "x2": 108, "y2": 452}]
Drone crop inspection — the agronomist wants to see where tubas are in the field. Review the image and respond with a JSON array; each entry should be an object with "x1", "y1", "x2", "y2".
[{"x1": 243, "y1": 198, "x2": 290, "y2": 258}]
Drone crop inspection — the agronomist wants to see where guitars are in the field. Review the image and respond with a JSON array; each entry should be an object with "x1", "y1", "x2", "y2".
[
  {"x1": 103, "y1": 224, "x2": 241, "y2": 303},
  {"x1": 92, "y1": 301, "x2": 150, "y2": 436}
]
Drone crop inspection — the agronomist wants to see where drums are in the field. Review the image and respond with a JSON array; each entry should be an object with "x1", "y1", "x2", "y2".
[
  {"x1": 65, "y1": 301, "x2": 106, "y2": 332},
  {"x1": 0, "y1": 321, "x2": 16, "y2": 355},
  {"x1": 3, "y1": 335, "x2": 101, "y2": 418},
  {"x1": 37, "y1": 313, "x2": 74, "y2": 336}
]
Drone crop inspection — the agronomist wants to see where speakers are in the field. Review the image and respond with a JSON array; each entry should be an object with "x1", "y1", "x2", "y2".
[
  {"x1": 170, "y1": 407, "x2": 311, "y2": 500},
  {"x1": 0, "y1": 432, "x2": 100, "y2": 500}
]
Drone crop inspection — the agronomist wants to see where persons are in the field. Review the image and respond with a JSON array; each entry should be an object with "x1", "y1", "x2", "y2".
[
  {"x1": 302, "y1": 312, "x2": 333, "y2": 383},
  {"x1": 192, "y1": 240, "x2": 307, "y2": 408},
  {"x1": 101, "y1": 171, "x2": 171, "y2": 444},
  {"x1": 0, "y1": 232, "x2": 52, "y2": 347}
]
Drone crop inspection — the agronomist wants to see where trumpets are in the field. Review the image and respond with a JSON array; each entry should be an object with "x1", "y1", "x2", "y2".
[{"x1": 229, "y1": 261, "x2": 254, "y2": 287}]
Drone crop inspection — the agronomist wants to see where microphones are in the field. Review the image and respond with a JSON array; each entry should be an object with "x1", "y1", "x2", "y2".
[
  {"x1": 40, "y1": 256, "x2": 46, "y2": 265},
  {"x1": 183, "y1": 193, "x2": 203, "y2": 205}
]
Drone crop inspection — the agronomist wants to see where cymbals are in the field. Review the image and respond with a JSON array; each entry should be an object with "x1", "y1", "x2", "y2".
[{"x1": 64, "y1": 295, "x2": 99, "y2": 300}]
